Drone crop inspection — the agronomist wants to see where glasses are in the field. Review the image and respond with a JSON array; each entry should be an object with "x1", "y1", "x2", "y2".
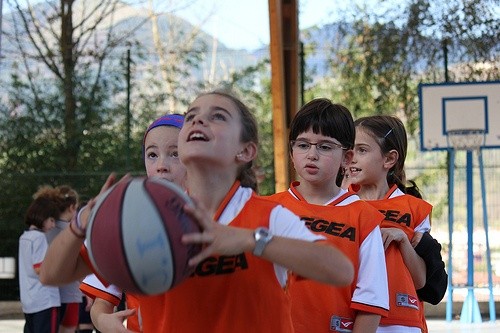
[{"x1": 290, "y1": 139, "x2": 350, "y2": 154}]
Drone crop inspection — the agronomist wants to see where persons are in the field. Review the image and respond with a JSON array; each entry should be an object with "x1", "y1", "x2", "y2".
[
  {"x1": 18, "y1": 196, "x2": 61, "y2": 333},
  {"x1": 345, "y1": 116, "x2": 448, "y2": 333},
  {"x1": 78, "y1": 294, "x2": 94, "y2": 333},
  {"x1": 79, "y1": 114, "x2": 206, "y2": 333},
  {"x1": 40, "y1": 90, "x2": 355, "y2": 333},
  {"x1": 261, "y1": 99, "x2": 389, "y2": 333},
  {"x1": 31, "y1": 186, "x2": 81, "y2": 333}
]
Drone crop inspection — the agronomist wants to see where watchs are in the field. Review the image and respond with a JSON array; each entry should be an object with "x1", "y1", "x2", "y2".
[{"x1": 252, "y1": 226, "x2": 272, "y2": 257}]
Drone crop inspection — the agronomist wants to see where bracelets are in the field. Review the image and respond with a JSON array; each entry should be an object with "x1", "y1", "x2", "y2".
[{"x1": 70, "y1": 205, "x2": 87, "y2": 240}]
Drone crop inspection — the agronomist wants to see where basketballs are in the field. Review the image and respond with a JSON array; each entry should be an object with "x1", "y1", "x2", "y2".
[{"x1": 86, "y1": 177, "x2": 204, "y2": 296}]
